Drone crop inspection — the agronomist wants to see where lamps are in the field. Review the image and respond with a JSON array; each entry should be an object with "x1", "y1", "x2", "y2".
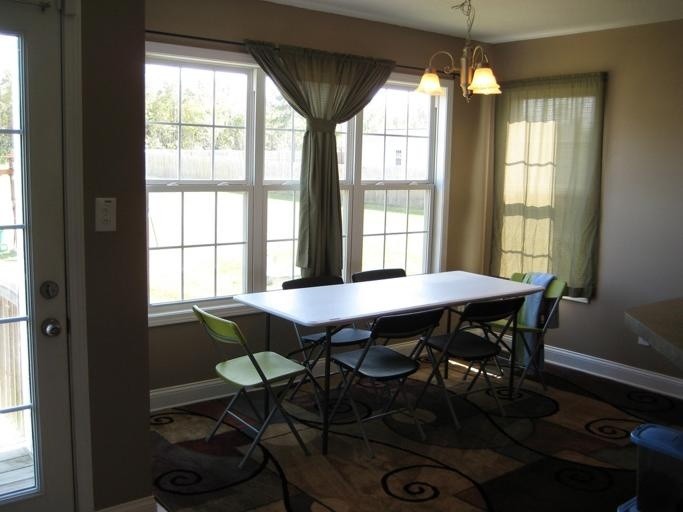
[{"x1": 411, "y1": 0, "x2": 503, "y2": 103}]
